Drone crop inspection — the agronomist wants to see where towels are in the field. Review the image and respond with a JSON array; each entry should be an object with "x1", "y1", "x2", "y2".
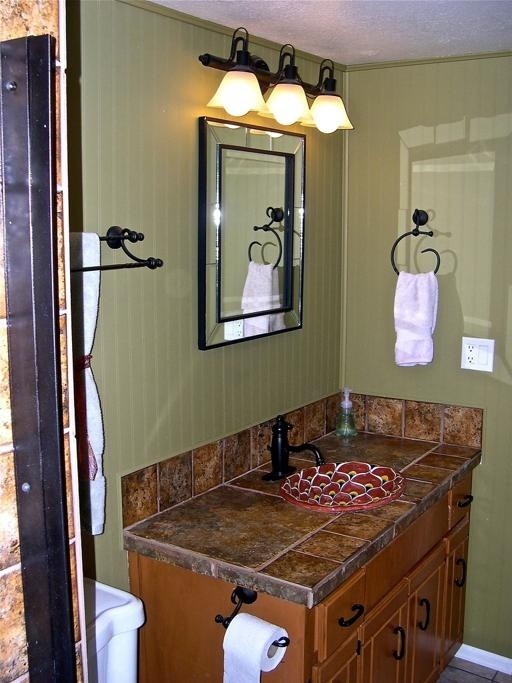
[
  {"x1": 394, "y1": 270, "x2": 438, "y2": 367},
  {"x1": 82, "y1": 233, "x2": 106, "y2": 536},
  {"x1": 241, "y1": 261, "x2": 286, "y2": 333}
]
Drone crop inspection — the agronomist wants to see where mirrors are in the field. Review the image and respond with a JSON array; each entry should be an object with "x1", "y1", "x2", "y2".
[{"x1": 198, "y1": 116, "x2": 307, "y2": 350}]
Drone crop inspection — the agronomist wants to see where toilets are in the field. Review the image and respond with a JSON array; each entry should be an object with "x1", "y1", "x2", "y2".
[{"x1": 79, "y1": 574, "x2": 145, "y2": 683}]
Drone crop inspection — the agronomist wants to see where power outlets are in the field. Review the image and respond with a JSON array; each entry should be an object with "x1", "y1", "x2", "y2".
[{"x1": 460, "y1": 337, "x2": 495, "y2": 373}]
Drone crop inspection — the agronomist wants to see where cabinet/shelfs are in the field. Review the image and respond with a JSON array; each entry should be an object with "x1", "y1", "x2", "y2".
[{"x1": 128, "y1": 471, "x2": 473, "y2": 682}]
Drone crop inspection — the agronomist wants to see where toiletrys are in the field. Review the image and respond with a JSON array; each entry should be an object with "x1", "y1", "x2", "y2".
[{"x1": 335, "y1": 388, "x2": 361, "y2": 440}]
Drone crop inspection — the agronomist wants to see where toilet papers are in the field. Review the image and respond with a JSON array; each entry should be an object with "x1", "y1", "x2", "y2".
[{"x1": 220, "y1": 612, "x2": 290, "y2": 683}]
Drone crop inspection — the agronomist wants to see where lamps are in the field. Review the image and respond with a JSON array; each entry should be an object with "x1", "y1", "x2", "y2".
[{"x1": 199, "y1": 26, "x2": 354, "y2": 133}]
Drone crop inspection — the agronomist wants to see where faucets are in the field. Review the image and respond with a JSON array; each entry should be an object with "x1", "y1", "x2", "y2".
[{"x1": 260, "y1": 413, "x2": 327, "y2": 483}]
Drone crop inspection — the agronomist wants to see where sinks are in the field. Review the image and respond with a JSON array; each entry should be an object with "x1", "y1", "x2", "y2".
[{"x1": 278, "y1": 458, "x2": 409, "y2": 514}]
